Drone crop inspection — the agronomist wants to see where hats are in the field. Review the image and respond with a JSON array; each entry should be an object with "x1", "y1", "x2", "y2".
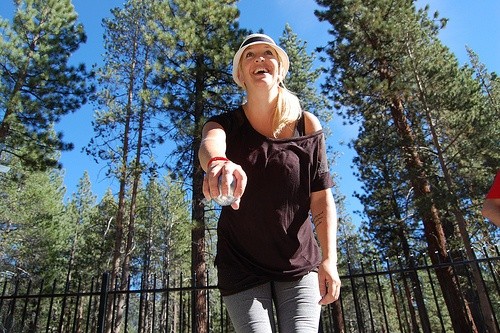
[{"x1": 232, "y1": 33, "x2": 290, "y2": 89}]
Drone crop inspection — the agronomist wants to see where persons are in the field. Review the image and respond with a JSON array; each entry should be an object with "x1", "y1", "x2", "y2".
[
  {"x1": 479, "y1": 169, "x2": 500, "y2": 228},
  {"x1": 196, "y1": 33, "x2": 342, "y2": 333}
]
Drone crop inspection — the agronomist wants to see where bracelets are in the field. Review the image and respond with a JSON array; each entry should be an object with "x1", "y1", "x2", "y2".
[{"x1": 206, "y1": 156, "x2": 230, "y2": 171}]
